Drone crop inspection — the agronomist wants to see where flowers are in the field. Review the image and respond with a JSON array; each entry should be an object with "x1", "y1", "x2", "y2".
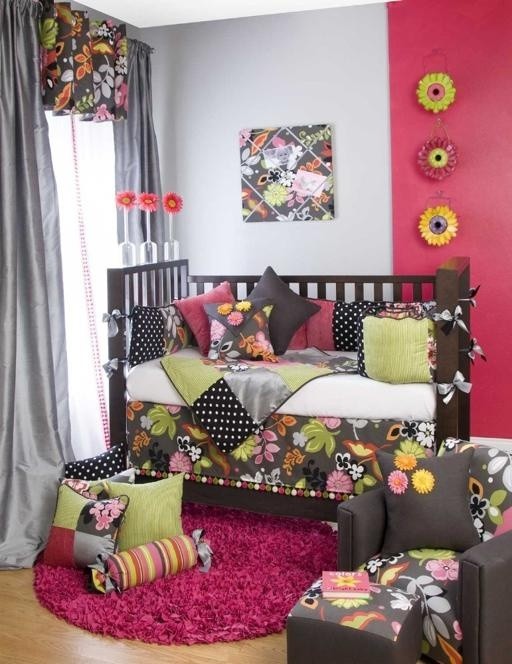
[
  {"x1": 216, "y1": 299, "x2": 252, "y2": 330},
  {"x1": 162, "y1": 189, "x2": 183, "y2": 215},
  {"x1": 87, "y1": 498, "x2": 127, "y2": 532},
  {"x1": 137, "y1": 190, "x2": 160, "y2": 213},
  {"x1": 114, "y1": 188, "x2": 136, "y2": 208},
  {"x1": 415, "y1": 70, "x2": 460, "y2": 248},
  {"x1": 387, "y1": 454, "x2": 438, "y2": 498}
]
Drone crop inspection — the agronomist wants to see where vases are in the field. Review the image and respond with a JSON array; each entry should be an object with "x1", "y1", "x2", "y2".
[
  {"x1": 118, "y1": 206, "x2": 137, "y2": 268},
  {"x1": 139, "y1": 209, "x2": 158, "y2": 264},
  {"x1": 163, "y1": 210, "x2": 182, "y2": 262}
]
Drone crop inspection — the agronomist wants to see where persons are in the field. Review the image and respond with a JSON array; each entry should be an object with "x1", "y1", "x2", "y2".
[{"x1": 275, "y1": 147, "x2": 293, "y2": 172}]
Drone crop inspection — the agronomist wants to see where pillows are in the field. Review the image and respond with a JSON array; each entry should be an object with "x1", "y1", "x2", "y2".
[
  {"x1": 174, "y1": 267, "x2": 436, "y2": 384},
  {"x1": 98, "y1": 467, "x2": 190, "y2": 553},
  {"x1": 62, "y1": 440, "x2": 125, "y2": 478},
  {"x1": 37, "y1": 480, "x2": 132, "y2": 575},
  {"x1": 86, "y1": 525, "x2": 216, "y2": 595},
  {"x1": 372, "y1": 445, "x2": 482, "y2": 555},
  {"x1": 109, "y1": 466, "x2": 137, "y2": 486}
]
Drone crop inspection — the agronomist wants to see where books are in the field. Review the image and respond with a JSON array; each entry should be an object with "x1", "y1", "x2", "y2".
[{"x1": 321, "y1": 570, "x2": 371, "y2": 599}]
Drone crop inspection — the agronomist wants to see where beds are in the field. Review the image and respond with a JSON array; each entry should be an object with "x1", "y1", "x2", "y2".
[{"x1": 101, "y1": 252, "x2": 473, "y2": 526}]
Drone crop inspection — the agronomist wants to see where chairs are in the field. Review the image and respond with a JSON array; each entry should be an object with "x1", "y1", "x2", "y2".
[{"x1": 282, "y1": 437, "x2": 512, "y2": 664}]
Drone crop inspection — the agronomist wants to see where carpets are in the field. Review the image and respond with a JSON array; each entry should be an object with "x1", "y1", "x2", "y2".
[{"x1": 32, "y1": 501, "x2": 340, "y2": 648}]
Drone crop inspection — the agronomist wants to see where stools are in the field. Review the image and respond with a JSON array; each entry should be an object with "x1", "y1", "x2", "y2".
[{"x1": 285, "y1": 574, "x2": 424, "y2": 664}]
